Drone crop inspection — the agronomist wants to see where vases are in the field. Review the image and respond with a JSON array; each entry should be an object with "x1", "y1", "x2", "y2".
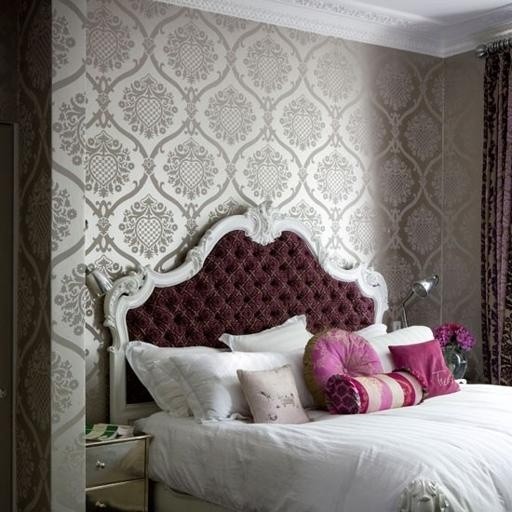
[{"x1": 444, "y1": 350, "x2": 468, "y2": 381}]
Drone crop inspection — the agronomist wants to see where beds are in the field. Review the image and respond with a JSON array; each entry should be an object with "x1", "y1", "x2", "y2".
[{"x1": 99, "y1": 195, "x2": 511, "y2": 507}]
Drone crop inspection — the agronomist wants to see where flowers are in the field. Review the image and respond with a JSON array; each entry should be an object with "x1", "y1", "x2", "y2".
[{"x1": 429, "y1": 321, "x2": 476, "y2": 359}]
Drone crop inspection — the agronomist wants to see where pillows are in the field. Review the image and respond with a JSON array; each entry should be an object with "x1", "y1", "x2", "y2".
[
  {"x1": 389, "y1": 339, "x2": 460, "y2": 397},
  {"x1": 154, "y1": 350, "x2": 313, "y2": 424},
  {"x1": 125, "y1": 341, "x2": 226, "y2": 417},
  {"x1": 354, "y1": 323, "x2": 387, "y2": 339},
  {"x1": 237, "y1": 365, "x2": 310, "y2": 425},
  {"x1": 219, "y1": 314, "x2": 316, "y2": 362},
  {"x1": 367, "y1": 325, "x2": 435, "y2": 373},
  {"x1": 325, "y1": 365, "x2": 427, "y2": 414},
  {"x1": 302, "y1": 328, "x2": 385, "y2": 412}
]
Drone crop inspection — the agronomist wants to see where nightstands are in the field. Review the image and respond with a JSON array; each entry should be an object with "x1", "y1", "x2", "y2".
[{"x1": 87, "y1": 430, "x2": 154, "y2": 512}]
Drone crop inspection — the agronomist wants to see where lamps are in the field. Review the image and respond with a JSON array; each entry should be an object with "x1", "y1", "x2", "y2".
[{"x1": 397, "y1": 271, "x2": 440, "y2": 329}]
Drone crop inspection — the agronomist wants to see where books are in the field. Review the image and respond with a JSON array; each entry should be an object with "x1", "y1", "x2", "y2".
[{"x1": 83, "y1": 423, "x2": 136, "y2": 441}]
